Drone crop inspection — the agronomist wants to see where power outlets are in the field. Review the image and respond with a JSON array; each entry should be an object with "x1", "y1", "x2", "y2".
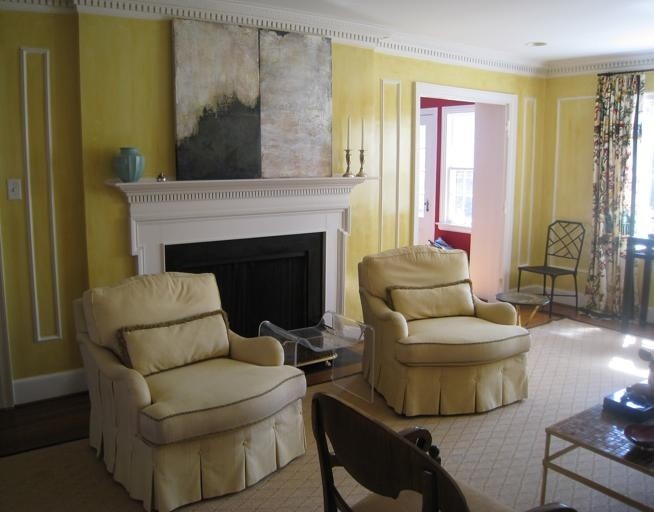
[{"x1": 7, "y1": 178, "x2": 22, "y2": 200}]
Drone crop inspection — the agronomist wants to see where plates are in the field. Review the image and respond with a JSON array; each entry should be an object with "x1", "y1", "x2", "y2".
[{"x1": 624, "y1": 423, "x2": 654, "y2": 448}]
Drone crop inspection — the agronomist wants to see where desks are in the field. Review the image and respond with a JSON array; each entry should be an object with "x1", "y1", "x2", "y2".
[{"x1": 539, "y1": 378, "x2": 654, "y2": 512}]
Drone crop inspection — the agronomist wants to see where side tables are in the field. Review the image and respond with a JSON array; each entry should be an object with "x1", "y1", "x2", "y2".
[
  {"x1": 258, "y1": 310, "x2": 376, "y2": 406},
  {"x1": 496, "y1": 291, "x2": 551, "y2": 328}
]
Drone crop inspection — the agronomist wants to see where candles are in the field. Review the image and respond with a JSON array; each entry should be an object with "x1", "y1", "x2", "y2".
[
  {"x1": 360, "y1": 117, "x2": 365, "y2": 150},
  {"x1": 346, "y1": 116, "x2": 350, "y2": 150}
]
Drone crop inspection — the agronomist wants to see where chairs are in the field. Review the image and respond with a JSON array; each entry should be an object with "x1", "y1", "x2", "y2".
[
  {"x1": 311, "y1": 392, "x2": 580, "y2": 511},
  {"x1": 70, "y1": 271, "x2": 307, "y2": 512},
  {"x1": 517, "y1": 220, "x2": 585, "y2": 320},
  {"x1": 358, "y1": 244, "x2": 531, "y2": 418}
]
